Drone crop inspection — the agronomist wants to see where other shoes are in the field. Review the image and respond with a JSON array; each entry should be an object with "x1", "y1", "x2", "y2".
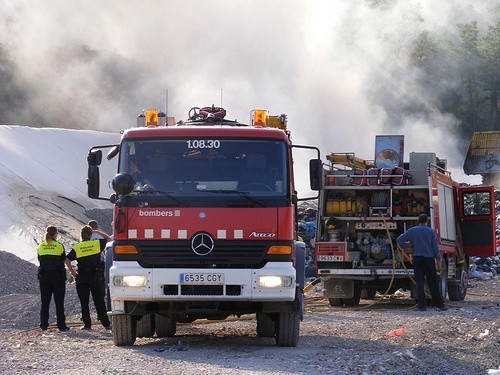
[
  {"x1": 105, "y1": 325, "x2": 112, "y2": 331},
  {"x1": 80, "y1": 325, "x2": 91, "y2": 330},
  {"x1": 60, "y1": 326, "x2": 71, "y2": 331},
  {"x1": 412, "y1": 304, "x2": 427, "y2": 311},
  {"x1": 41, "y1": 327, "x2": 48, "y2": 331},
  {"x1": 436, "y1": 304, "x2": 449, "y2": 311}
]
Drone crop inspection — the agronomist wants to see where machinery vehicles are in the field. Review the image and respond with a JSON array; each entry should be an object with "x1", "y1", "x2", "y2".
[
  {"x1": 86, "y1": 106, "x2": 323, "y2": 347},
  {"x1": 314, "y1": 133, "x2": 496, "y2": 308}
]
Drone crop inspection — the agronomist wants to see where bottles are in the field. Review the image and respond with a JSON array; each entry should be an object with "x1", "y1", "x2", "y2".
[{"x1": 386, "y1": 328, "x2": 406, "y2": 336}]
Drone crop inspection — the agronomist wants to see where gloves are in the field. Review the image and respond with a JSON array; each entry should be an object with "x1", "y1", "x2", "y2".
[
  {"x1": 405, "y1": 247, "x2": 414, "y2": 254},
  {"x1": 437, "y1": 259, "x2": 442, "y2": 272}
]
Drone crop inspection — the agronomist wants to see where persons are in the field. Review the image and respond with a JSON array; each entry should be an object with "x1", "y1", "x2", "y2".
[
  {"x1": 66, "y1": 226, "x2": 112, "y2": 330},
  {"x1": 397, "y1": 214, "x2": 445, "y2": 311},
  {"x1": 37, "y1": 225, "x2": 70, "y2": 331},
  {"x1": 88, "y1": 220, "x2": 113, "y2": 278}
]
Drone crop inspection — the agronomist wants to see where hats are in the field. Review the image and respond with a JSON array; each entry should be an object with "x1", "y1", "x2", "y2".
[{"x1": 88, "y1": 220, "x2": 100, "y2": 229}]
